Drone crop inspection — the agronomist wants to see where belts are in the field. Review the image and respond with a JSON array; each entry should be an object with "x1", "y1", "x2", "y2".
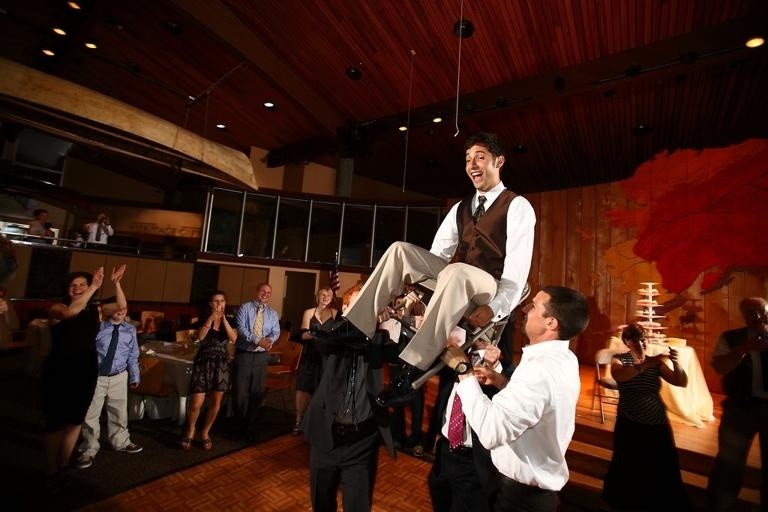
[{"x1": 335, "y1": 421, "x2": 379, "y2": 435}]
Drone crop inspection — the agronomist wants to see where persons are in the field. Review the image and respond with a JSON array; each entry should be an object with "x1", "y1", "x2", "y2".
[
  {"x1": 704, "y1": 295, "x2": 768, "y2": 510},
  {"x1": 24, "y1": 207, "x2": 115, "y2": 251},
  {"x1": 181, "y1": 289, "x2": 239, "y2": 452},
  {"x1": 603, "y1": 321, "x2": 688, "y2": 512},
  {"x1": 293, "y1": 285, "x2": 425, "y2": 512},
  {"x1": 441, "y1": 285, "x2": 592, "y2": 511},
  {"x1": 38, "y1": 263, "x2": 129, "y2": 476},
  {"x1": 307, "y1": 133, "x2": 538, "y2": 407},
  {"x1": 1, "y1": 236, "x2": 38, "y2": 351},
  {"x1": 232, "y1": 281, "x2": 282, "y2": 442},
  {"x1": 292, "y1": 285, "x2": 515, "y2": 460},
  {"x1": 75, "y1": 295, "x2": 144, "y2": 470}
]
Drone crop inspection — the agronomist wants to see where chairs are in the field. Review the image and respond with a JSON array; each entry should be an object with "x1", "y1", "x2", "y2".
[
  {"x1": 168, "y1": 307, "x2": 194, "y2": 332},
  {"x1": 377, "y1": 283, "x2": 532, "y2": 389},
  {"x1": 591, "y1": 347, "x2": 621, "y2": 425},
  {"x1": 138, "y1": 309, "x2": 164, "y2": 338},
  {"x1": 262, "y1": 340, "x2": 303, "y2": 414}
]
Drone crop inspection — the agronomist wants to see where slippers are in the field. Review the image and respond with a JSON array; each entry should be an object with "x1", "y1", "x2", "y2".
[
  {"x1": 62, "y1": 471, "x2": 71, "y2": 492},
  {"x1": 46, "y1": 471, "x2": 60, "y2": 493}
]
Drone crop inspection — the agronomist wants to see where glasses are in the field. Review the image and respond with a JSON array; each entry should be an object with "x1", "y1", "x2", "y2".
[{"x1": 744, "y1": 312, "x2": 766, "y2": 319}]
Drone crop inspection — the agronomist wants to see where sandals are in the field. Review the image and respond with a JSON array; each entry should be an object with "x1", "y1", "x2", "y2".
[
  {"x1": 182, "y1": 428, "x2": 196, "y2": 449},
  {"x1": 200, "y1": 435, "x2": 212, "y2": 450}
]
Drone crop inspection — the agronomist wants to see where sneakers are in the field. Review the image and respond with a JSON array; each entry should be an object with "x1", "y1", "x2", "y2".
[
  {"x1": 76, "y1": 455, "x2": 93, "y2": 469},
  {"x1": 292, "y1": 422, "x2": 304, "y2": 435},
  {"x1": 118, "y1": 443, "x2": 142, "y2": 453},
  {"x1": 413, "y1": 445, "x2": 424, "y2": 457}
]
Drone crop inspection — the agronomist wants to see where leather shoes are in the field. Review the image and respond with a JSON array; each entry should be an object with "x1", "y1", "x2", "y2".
[
  {"x1": 310, "y1": 323, "x2": 363, "y2": 345},
  {"x1": 377, "y1": 385, "x2": 415, "y2": 409}
]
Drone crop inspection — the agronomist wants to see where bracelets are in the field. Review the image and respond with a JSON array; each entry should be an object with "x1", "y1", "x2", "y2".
[
  {"x1": 202, "y1": 323, "x2": 212, "y2": 331},
  {"x1": 636, "y1": 363, "x2": 645, "y2": 376}
]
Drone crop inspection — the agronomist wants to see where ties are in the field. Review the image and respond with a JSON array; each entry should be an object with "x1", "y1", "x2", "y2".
[
  {"x1": 472, "y1": 196, "x2": 487, "y2": 223},
  {"x1": 448, "y1": 393, "x2": 464, "y2": 450},
  {"x1": 253, "y1": 305, "x2": 264, "y2": 338},
  {"x1": 101, "y1": 325, "x2": 119, "y2": 375},
  {"x1": 95, "y1": 222, "x2": 102, "y2": 241}
]
